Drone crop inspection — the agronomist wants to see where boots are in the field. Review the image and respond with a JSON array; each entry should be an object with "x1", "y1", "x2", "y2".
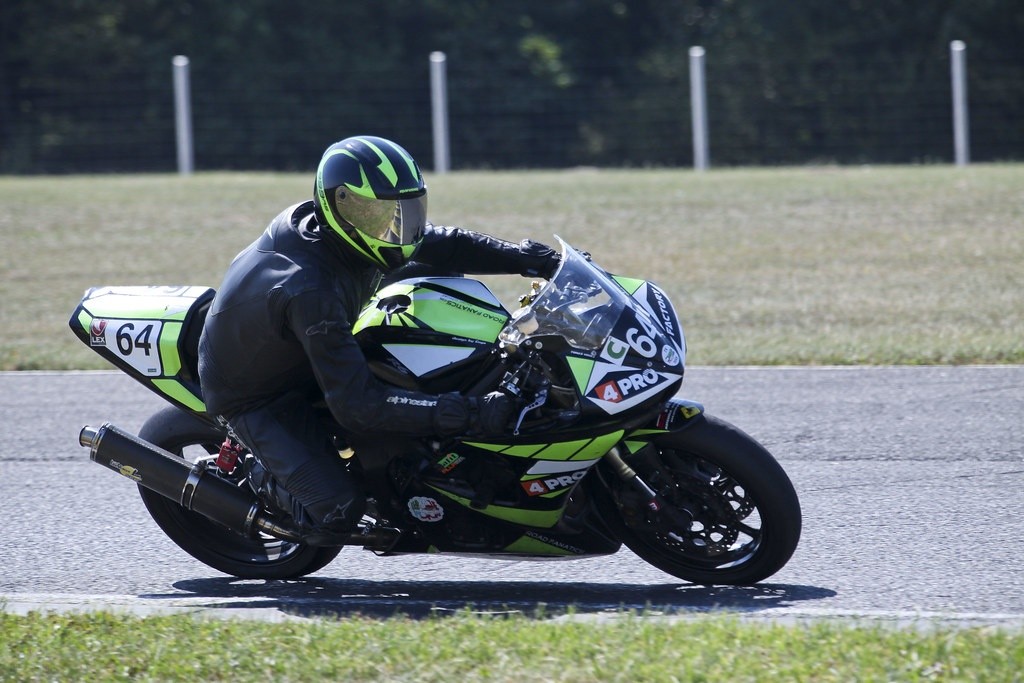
[{"x1": 244, "y1": 455, "x2": 311, "y2": 540}]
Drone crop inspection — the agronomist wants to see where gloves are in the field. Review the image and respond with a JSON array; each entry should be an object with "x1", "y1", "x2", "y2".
[
  {"x1": 435, "y1": 392, "x2": 516, "y2": 441},
  {"x1": 520, "y1": 239, "x2": 591, "y2": 281}
]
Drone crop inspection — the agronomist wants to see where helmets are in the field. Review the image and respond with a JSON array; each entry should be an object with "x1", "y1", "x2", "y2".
[{"x1": 314, "y1": 136, "x2": 427, "y2": 274}]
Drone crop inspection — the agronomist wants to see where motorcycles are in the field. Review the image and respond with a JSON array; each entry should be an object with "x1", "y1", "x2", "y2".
[{"x1": 68, "y1": 231, "x2": 800, "y2": 591}]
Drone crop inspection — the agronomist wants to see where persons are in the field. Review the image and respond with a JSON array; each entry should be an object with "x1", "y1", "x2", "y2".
[{"x1": 196, "y1": 134, "x2": 614, "y2": 535}]
